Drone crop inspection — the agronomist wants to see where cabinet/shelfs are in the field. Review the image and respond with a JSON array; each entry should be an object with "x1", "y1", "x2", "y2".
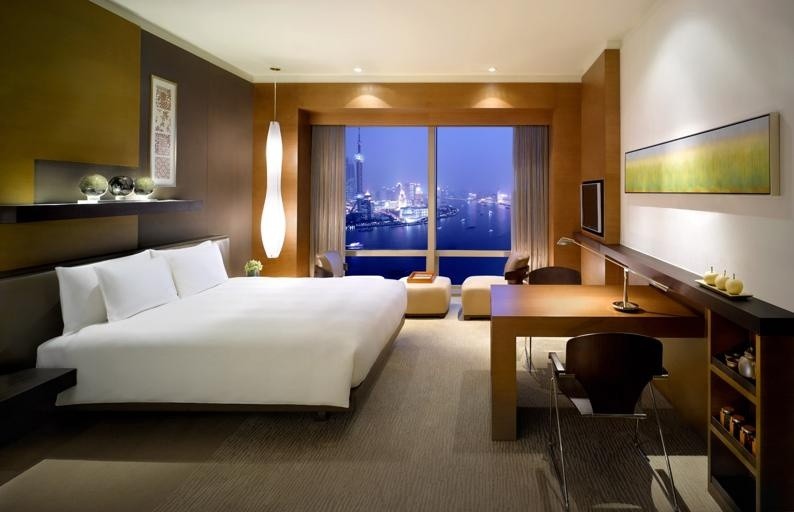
[
  {"x1": 1, "y1": 199, "x2": 204, "y2": 223},
  {"x1": 567, "y1": 233, "x2": 793, "y2": 512}
]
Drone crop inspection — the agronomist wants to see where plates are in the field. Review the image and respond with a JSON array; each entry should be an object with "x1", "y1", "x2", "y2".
[{"x1": 694, "y1": 278, "x2": 753, "y2": 299}]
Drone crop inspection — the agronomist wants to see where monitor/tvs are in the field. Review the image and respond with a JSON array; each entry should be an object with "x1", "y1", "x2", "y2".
[{"x1": 580, "y1": 183, "x2": 604, "y2": 234}]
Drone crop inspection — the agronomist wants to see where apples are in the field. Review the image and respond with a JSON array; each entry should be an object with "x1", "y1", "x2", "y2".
[
  {"x1": 704, "y1": 266, "x2": 720, "y2": 285},
  {"x1": 725, "y1": 273, "x2": 743, "y2": 294},
  {"x1": 715, "y1": 270, "x2": 730, "y2": 290}
]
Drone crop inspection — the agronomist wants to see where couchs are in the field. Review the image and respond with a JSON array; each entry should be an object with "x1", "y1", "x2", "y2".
[
  {"x1": 315, "y1": 252, "x2": 348, "y2": 278},
  {"x1": 462, "y1": 253, "x2": 531, "y2": 319},
  {"x1": 399, "y1": 276, "x2": 452, "y2": 318}
]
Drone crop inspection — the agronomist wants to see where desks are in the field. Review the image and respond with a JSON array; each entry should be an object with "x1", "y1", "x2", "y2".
[{"x1": 491, "y1": 284, "x2": 710, "y2": 442}]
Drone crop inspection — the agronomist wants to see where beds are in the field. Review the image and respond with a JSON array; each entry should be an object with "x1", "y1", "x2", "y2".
[{"x1": 35, "y1": 276, "x2": 407, "y2": 421}]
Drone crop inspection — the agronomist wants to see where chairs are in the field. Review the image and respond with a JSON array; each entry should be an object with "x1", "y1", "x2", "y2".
[
  {"x1": 549, "y1": 332, "x2": 689, "y2": 512},
  {"x1": 523, "y1": 267, "x2": 582, "y2": 374}
]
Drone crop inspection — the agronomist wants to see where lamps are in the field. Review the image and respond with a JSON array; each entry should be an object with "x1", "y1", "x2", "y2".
[
  {"x1": 260, "y1": 120, "x2": 286, "y2": 259},
  {"x1": 556, "y1": 237, "x2": 669, "y2": 311}
]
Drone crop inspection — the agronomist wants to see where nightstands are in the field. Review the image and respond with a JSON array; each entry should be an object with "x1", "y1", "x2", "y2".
[{"x1": 2, "y1": 368, "x2": 79, "y2": 445}]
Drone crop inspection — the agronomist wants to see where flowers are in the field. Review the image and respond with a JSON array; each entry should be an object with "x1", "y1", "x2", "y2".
[{"x1": 244, "y1": 260, "x2": 262, "y2": 277}]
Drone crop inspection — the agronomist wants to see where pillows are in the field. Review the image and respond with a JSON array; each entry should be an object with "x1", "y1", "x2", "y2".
[
  {"x1": 55, "y1": 249, "x2": 151, "y2": 336},
  {"x1": 163, "y1": 241, "x2": 228, "y2": 299},
  {"x1": 96, "y1": 254, "x2": 177, "y2": 322}
]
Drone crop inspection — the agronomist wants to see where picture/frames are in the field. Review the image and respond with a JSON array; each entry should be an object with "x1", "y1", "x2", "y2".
[
  {"x1": 625, "y1": 112, "x2": 780, "y2": 197},
  {"x1": 150, "y1": 74, "x2": 178, "y2": 188}
]
